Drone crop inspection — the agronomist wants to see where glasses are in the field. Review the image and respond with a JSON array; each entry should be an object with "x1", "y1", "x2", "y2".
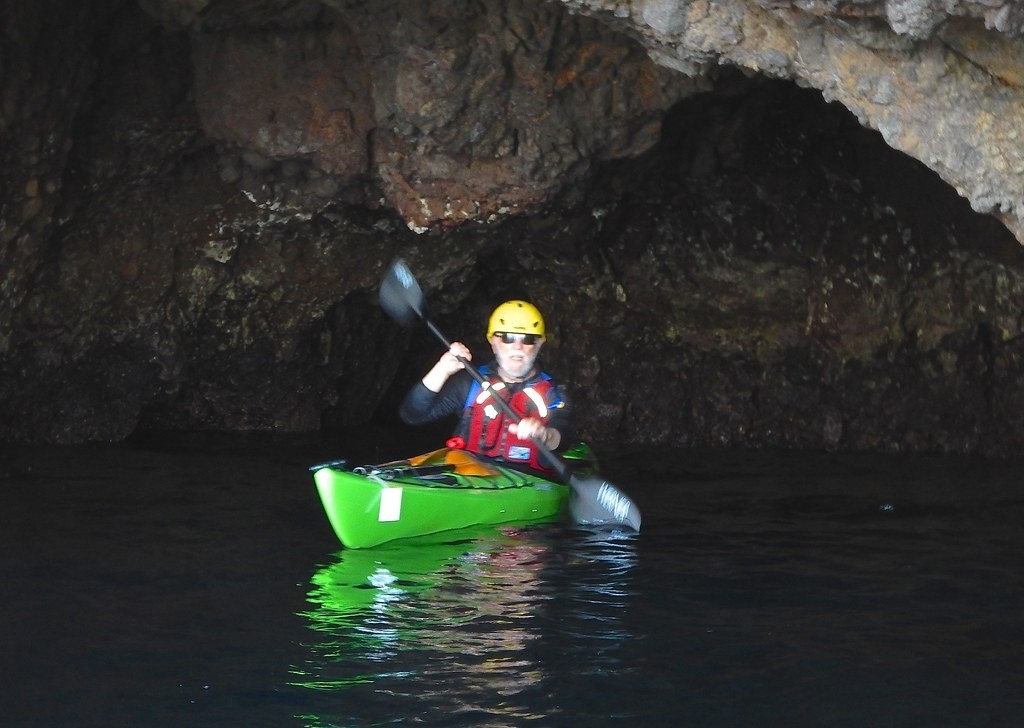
[{"x1": 493, "y1": 332, "x2": 537, "y2": 345}]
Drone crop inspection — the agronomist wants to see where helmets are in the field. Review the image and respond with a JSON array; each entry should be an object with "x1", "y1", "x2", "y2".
[{"x1": 487, "y1": 300, "x2": 547, "y2": 343}]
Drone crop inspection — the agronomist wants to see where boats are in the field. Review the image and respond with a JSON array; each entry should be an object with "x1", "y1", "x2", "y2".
[{"x1": 309, "y1": 430, "x2": 601, "y2": 547}]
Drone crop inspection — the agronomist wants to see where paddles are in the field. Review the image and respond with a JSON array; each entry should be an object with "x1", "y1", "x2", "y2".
[{"x1": 377, "y1": 257, "x2": 643, "y2": 535}]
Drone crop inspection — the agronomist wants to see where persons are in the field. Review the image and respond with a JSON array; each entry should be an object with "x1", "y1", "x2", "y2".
[{"x1": 396, "y1": 299, "x2": 574, "y2": 475}]
